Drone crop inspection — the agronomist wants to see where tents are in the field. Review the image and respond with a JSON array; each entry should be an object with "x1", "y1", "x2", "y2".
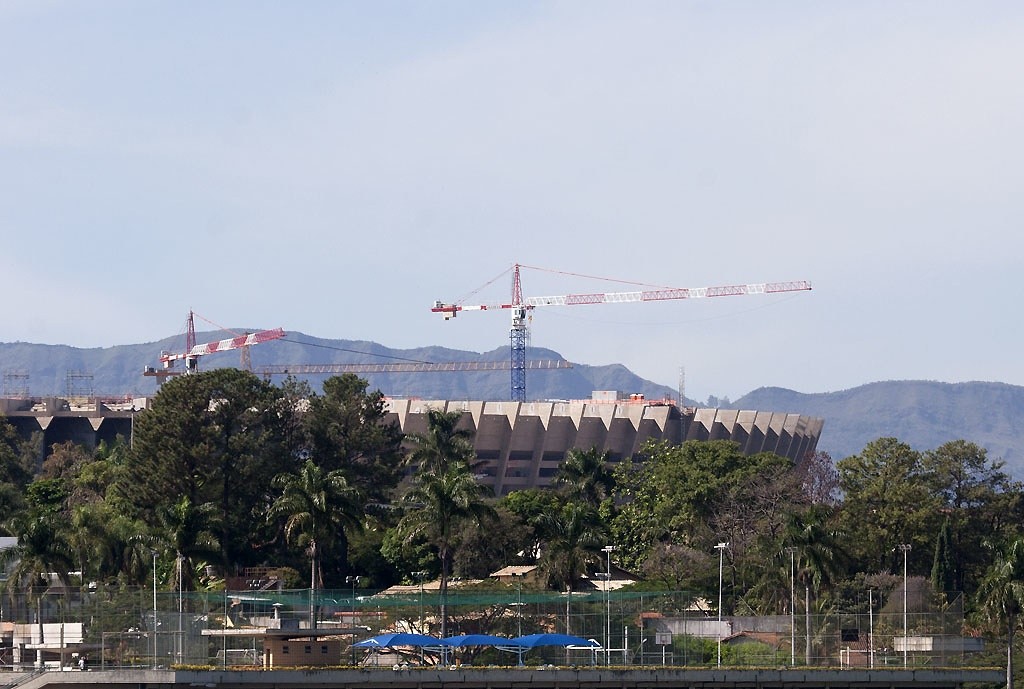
[{"x1": 351, "y1": 633, "x2": 600, "y2": 667}]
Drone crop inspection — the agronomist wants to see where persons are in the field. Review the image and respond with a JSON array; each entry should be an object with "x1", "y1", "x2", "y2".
[
  {"x1": 451, "y1": 635, "x2": 466, "y2": 668},
  {"x1": 78, "y1": 656, "x2": 88, "y2": 671}
]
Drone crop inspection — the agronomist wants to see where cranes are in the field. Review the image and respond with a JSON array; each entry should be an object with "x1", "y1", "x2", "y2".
[
  {"x1": 145, "y1": 358, "x2": 573, "y2": 388},
  {"x1": 433, "y1": 263, "x2": 812, "y2": 403},
  {"x1": 159, "y1": 309, "x2": 286, "y2": 371}
]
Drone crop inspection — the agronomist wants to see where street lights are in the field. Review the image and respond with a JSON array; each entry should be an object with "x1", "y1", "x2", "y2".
[
  {"x1": 411, "y1": 572, "x2": 429, "y2": 666},
  {"x1": 714, "y1": 541, "x2": 729, "y2": 667},
  {"x1": 600, "y1": 545, "x2": 617, "y2": 665},
  {"x1": 898, "y1": 544, "x2": 912, "y2": 667},
  {"x1": 512, "y1": 572, "x2": 527, "y2": 666},
  {"x1": 785, "y1": 546, "x2": 799, "y2": 665},
  {"x1": 346, "y1": 575, "x2": 362, "y2": 666},
  {"x1": 153, "y1": 553, "x2": 159, "y2": 667},
  {"x1": 175, "y1": 552, "x2": 185, "y2": 662}
]
